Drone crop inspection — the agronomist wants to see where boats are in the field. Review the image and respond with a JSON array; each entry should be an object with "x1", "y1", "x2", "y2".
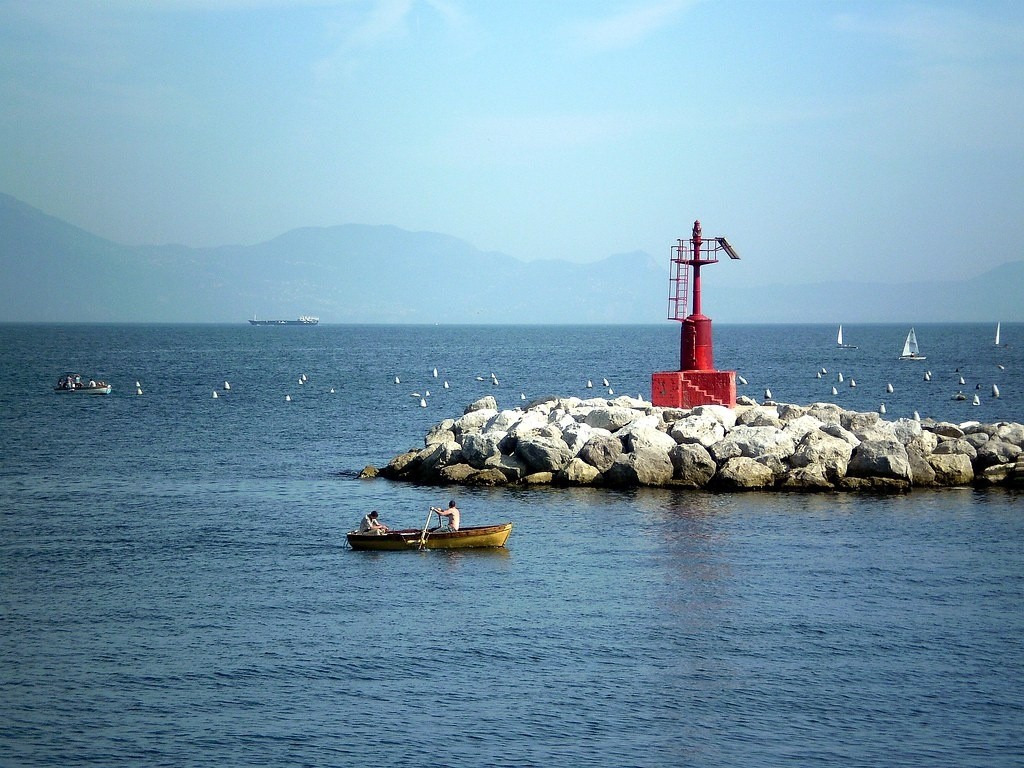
[
  {"x1": 54, "y1": 384, "x2": 112, "y2": 394},
  {"x1": 248, "y1": 313, "x2": 319, "y2": 326},
  {"x1": 346, "y1": 507, "x2": 513, "y2": 550}
]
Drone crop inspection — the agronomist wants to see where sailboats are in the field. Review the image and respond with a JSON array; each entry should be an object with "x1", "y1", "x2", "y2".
[
  {"x1": 836, "y1": 324, "x2": 859, "y2": 350},
  {"x1": 898, "y1": 327, "x2": 926, "y2": 360},
  {"x1": 992, "y1": 320, "x2": 1009, "y2": 349}
]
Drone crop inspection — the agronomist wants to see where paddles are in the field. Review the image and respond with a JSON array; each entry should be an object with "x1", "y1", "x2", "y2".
[
  {"x1": 418, "y1": 510, "x2": 433, "y2": 550},
  {"x1": 439, "y1": 514, "x2": 443, "y2": 527}
]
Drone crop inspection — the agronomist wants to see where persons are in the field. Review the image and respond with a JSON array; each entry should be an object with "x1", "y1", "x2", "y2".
[
  {"x1": 359, "y1": 511, "x2": 387, "y2": 534},
  {"x1": 58, "y1": 374, "x2": 81, "y2": 389},
  {"x1": 431, "y1": 500, "x2": 460, "y2": 533},
  {"x1": 89, "y1": 379, "x2": 96, "y2": 388},
  {"x1": 911, "y1": 352, "x2": 915, "y2": 357}
]
